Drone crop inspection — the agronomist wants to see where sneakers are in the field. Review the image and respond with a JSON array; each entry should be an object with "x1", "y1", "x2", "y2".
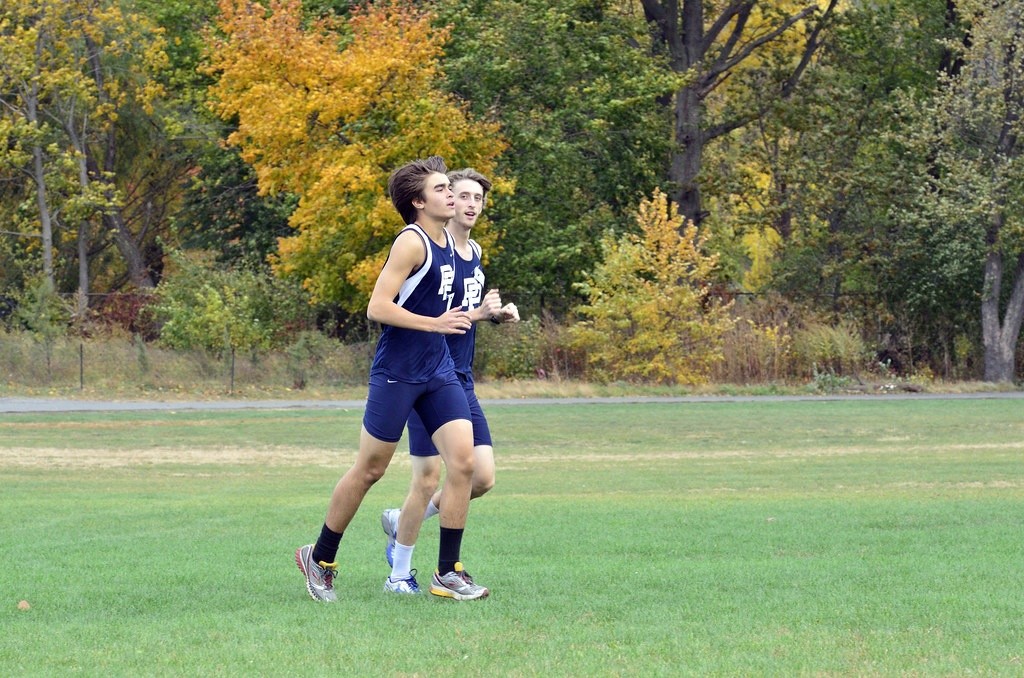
[
  {"x1": 383, "y1": 569, "x2": 423, "y2": 594},
  {"x1": 382, "y1": 509, "x2": 401, "y2": 567},
  {"x1": 430, "y1": 562, "x2": 489, "y2": 601},
  {"x1": 296, "y1": 544, "x2": 338, "y2": 603}
]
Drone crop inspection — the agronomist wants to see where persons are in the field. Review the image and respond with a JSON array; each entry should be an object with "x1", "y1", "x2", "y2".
[
  {"x1": 295, "y1": 155, "x2": 490, "y2": 604},
  {"x1": 380, "y1": 166, "x2": 520, "y2": 595}
]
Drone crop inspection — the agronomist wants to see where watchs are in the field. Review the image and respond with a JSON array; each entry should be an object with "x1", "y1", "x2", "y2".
[{"x1": 491, "y1": 316, "x2": 500, "y2": 325}]
